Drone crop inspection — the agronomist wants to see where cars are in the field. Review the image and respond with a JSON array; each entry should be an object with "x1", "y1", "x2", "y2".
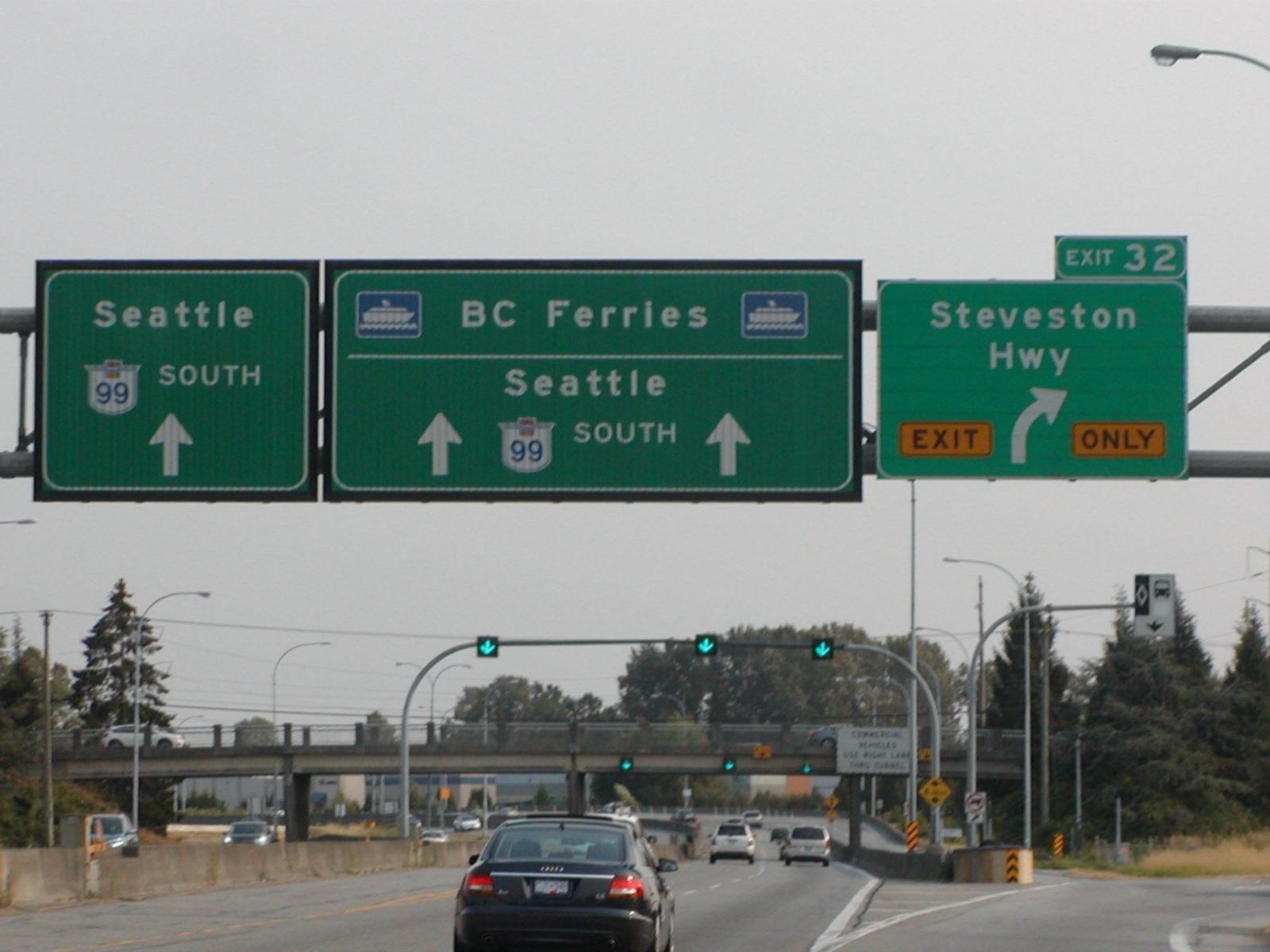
[
  {"x1": 452, "y1": 814, "x2": 482, "y2": 832},
  {"x1": 452, "y1": 798, "x2": 679, "y2": 952},
  {"x1": 808, "y1": 722, "x2": 853, "y2": 755},
  {"x1": 769, "y1": 822, "x2": 832, "y2": 867},
  {"x1": 420, "y1": 828, "x2": 450, "y2": 845},
  {"x1": 671, "y1": 807, "x2": 701, "y2": 825},
  {"x1": 706, "y1": 823, "x2": 758, "y2": 864},
  {"x1": 98, "y1": 720, "x2": 186, "y2": 753},
  {"x1": 222, "y1": 820, "x2": 272, "y2": 847},
  {"x1": 739, "y1": 809, "x2": 764, "y2": 829},
  {"x1": 83, "y1": 812, "x2": 140, "y2": 859}
]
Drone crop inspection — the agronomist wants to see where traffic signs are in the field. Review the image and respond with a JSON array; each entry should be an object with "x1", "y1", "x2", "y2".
[
  {"x1": 320, "y1": 257, "x2": 866, "y2": 506},
  {"x1": 477, "y1": 636, "x2": 498, "y2": 658},
  {"x1": 877, "y1": 233, "x2": 1192, "y2": 483},
  {"x1": 30, "y1": 259, "x2": 320, "y2": 505}
]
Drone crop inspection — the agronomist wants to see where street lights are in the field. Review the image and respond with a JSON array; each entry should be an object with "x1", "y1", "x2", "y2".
[
  {"x1": 130, "y1": 590, "x2": 213, "y2": 832},
  {"x1": 395, "y1": 661, "x2": 473, "y2": 745},
  {"x1": 270, "y1": 641, "x2": 331, "y2": 747},
  {"x1": 942, "y1": 556, "x2": 1033, "y2": 849},
  {"x1": 483, "y1": 679, "x2": 521, "y2": 745}
]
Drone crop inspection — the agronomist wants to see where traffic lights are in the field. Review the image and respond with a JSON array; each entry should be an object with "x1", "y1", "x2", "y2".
[
  {"x1": 810, "y1": 637, "x2": 835, "y2": 661},
  {"x1": 695, "y1": 633, "x2": 717, "y2": 656}
]
